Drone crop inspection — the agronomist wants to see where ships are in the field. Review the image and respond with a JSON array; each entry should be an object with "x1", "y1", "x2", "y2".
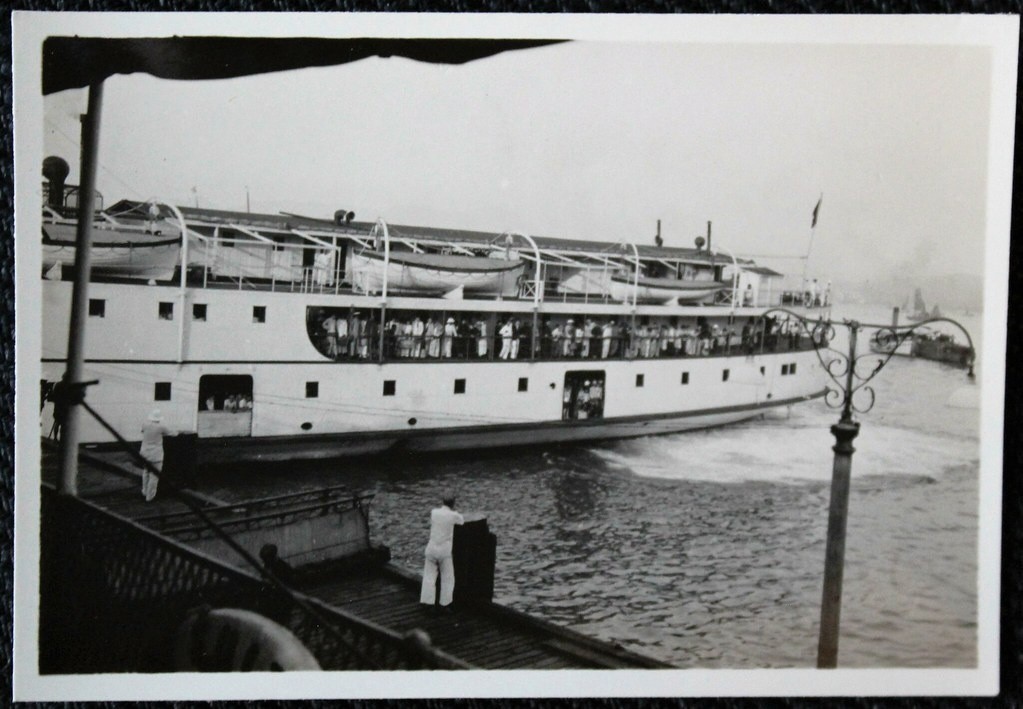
[{"x1": 41, "y1": 155, "x2": 835, "y2": 462}]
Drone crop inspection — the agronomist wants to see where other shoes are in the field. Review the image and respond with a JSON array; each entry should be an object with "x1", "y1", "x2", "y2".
[
  {"x1": 425, "y1": 605, "x2": 440, "y2": 619},
  {"x1": 438, "y1": 601, "x2": 461, "y2": 615}
]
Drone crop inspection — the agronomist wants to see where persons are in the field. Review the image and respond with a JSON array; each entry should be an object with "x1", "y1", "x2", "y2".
[
  {"x1": 743, "y1": 284, "x2": 753, "y2": 307},
  {"x1": 259, "y1": 543, "x2": 294, "y2": 588},
  {"x1": 314, "y1": 308, "x2": 831, "y2": 360},
  {"x1": 206, "y1": 390, "x2": 253, "y2": 410},
  {"x1": 418, "y1": 496, "x2": 463, "y2": 612},
  {"x1": 803, "y1": 280, "x2": 833, "y2": 308},
  {"x1": 139, "y1": 409, "x2": 178, "y2": 502},
  {"x1": 564, "y1": 377, "x2": 604, "y2": 422}
]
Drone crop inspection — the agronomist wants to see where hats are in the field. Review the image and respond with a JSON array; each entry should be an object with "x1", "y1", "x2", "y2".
[
  {"x1": 354, "y1": 312, "x2": 360, "y2": 315},
  {"x1": 447, "y1": 318, "x2": 455, "y2": 323},
  {"x1": 567, "y1": 318, "x2": 574, "y2": 324},
  {"x1": 148, "y1": 410, "x2": 164, "y2": 421}
]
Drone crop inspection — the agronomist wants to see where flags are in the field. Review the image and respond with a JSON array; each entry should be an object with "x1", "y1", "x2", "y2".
[{"x1": 811, "y1": 199, "x2": 821, "y2": 226}]
